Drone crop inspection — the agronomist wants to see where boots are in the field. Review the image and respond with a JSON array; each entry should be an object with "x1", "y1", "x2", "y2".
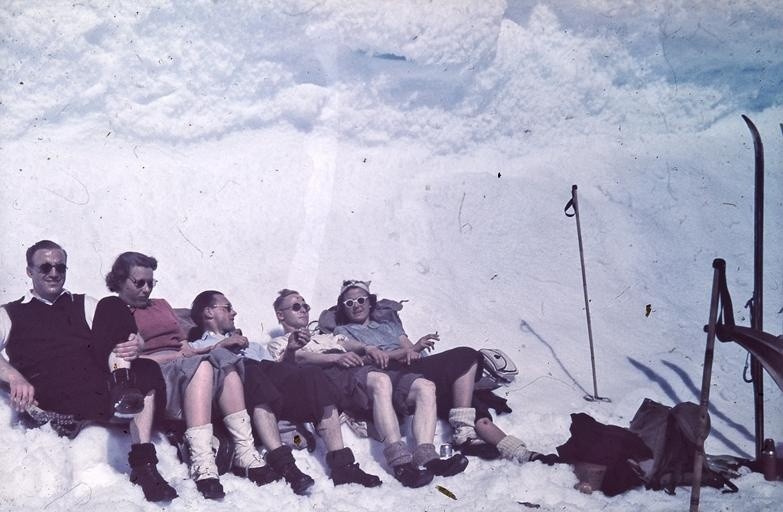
[{"x1": 184, "y1": 407, "x2": 278, "y2": 500}]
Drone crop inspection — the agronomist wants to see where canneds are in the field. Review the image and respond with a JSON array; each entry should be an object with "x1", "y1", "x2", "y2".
[{"x1": 440, "y1": 444, "x2": 452, "y2": 458}]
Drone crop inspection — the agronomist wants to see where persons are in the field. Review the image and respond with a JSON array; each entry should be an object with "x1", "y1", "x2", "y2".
[
  {"x1": 189, "y1": 288, "x2": 382, "y2": 494},
  {"x1": 265, "y1": 289, "x2": 468, "y2": 489},
  {"x1": 104, "y1": 253, "x2": 275, "y2": 497},
  {"x1": 0, "y1": 239, "x2": 177, "y2": 501},
  {"x1": 334, "y1": 279, "x2": 544, "y2": 464}
]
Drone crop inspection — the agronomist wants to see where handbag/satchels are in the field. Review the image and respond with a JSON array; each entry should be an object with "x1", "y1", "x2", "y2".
[{"x1": 475, "y1": 348, "x2": 518, "y2": 390}]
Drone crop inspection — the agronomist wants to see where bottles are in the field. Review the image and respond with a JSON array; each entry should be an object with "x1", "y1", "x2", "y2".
[{"x1": 760, "y1": 438, "x2": 778, "y2": 481}]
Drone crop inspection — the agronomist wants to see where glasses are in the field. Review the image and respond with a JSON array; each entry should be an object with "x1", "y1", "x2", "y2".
[
  {"x1": 280, "y1": 302, "x2": 310, "y2": 311},
  {"x1": 128, "y1": 273, "x2": 158, "y2": 287},
  {"x1": 213, "y1": 304, "x2": 231, "y2": 311},
  {"x1": 342, "y1": 297, "x2": 368, "y2": 308},
  {"x1": 30, "y1": 263, "x2": 66, "y2": 272}
]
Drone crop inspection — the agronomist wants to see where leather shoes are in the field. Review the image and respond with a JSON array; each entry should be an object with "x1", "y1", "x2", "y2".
[
  {"x1": 128, "y1": 463, "x2": 179, "y2": 501},
  {"x1": 398, "y1": 438, "x2": 500, "y2": 487},
  {"x1": 110, "y1": 379, "x2": 143, "y2": 425},
  {"x1": 326, "y1": 462, "x2": 383, "y2": 486},
  {"x1": 527, "y1": 450, "x2": 559, "y2": 464},
  {"x1": 274, "y1": 459, "x2": 313, "y2": 492}
]
